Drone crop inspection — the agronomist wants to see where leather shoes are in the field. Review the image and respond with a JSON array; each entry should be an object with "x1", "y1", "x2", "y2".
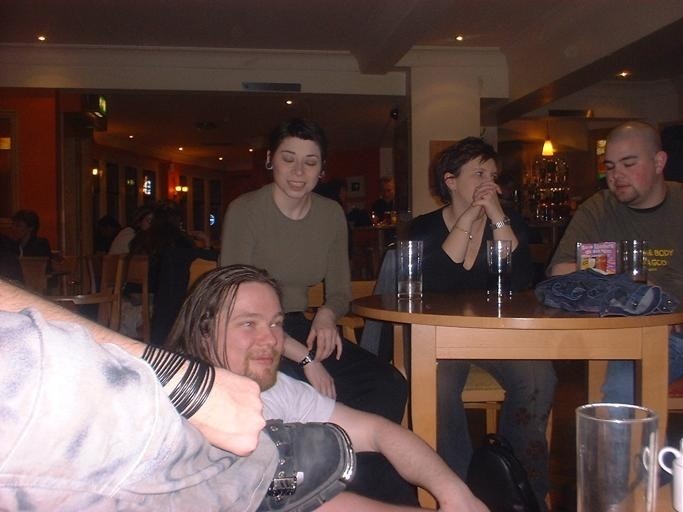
[{"x1": 255, "y1": 418, "x2": 358, "y2": 512}]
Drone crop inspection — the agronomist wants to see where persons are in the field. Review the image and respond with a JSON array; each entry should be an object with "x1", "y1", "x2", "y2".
[
  {"x1": 403, "y1": 136, "x2": 555, "y2": 512},
  {"x1": 316, "y1": 168, "x2": 397, "y2": 280},
  {"x1": 546, "y1": 119, "x2": 683, "y2": 511},
  {"x1": 0, "y1": 203, "x2": 218, "y2": 348},
  {"x1": 0, "y1": 277, "x2": 357, "y2": 512},
  {"x1": 219, "y1": 115, "x2": 406, "y2": 494},
  {"x1": 162, "y1": 263, "x2": 492, "y2": 512}
]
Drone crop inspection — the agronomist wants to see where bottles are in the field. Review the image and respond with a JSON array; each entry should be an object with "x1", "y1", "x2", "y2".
[
  {"x1": 368, "y1": 209, "x2": 400, "y2": 226},
  {"x1": 526, "y1": 159, "x2": 568, "y2": 220}
]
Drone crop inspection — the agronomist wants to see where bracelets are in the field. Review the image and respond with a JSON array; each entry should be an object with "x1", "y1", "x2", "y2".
[
  {"x1": 453, "y1": 224, "x2": 472, "y2": 239},
  {"x1": 141, "y1": 343, "x2": 215, "y2": 420},
  {"x1": 297, "y1": 350, "x2": 315, "y2": 369}
]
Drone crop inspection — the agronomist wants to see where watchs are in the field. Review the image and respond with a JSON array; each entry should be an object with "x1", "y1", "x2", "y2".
[{"x1": 489, "y1": 216, "x2": 511, "y2": 231}]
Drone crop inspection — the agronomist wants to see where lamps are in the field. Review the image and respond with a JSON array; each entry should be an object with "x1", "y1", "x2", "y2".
[{"x1": 542, "y1": 121, "x2": 554, "y2": 158}]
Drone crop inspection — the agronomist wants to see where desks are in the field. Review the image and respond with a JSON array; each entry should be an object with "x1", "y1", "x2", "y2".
[{"x1": 525, "y1": 223, "x2": 567, "y2": 270}]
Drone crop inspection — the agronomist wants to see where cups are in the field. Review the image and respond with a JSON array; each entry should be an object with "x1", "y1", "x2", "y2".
[
  {"x1": 395, "y1": 240, "x2": 423, "y2": 301},
  {"x1": 620, "y1": 240, "x2": 648, "y2": 283},
  {"x1": 575, "y1": 403, "x2": 658, "y2": 511},
  {"x1": 484, "y1": 241, "x2": 513, "y2": 297},
  {"x1": 657, "y1": 438, "x2": 681, "y2": 511}
]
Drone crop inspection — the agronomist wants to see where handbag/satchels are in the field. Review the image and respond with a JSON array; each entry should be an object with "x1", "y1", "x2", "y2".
[{"x1": 465, "y1": 434, "x2": 540, "y2": 512}]
